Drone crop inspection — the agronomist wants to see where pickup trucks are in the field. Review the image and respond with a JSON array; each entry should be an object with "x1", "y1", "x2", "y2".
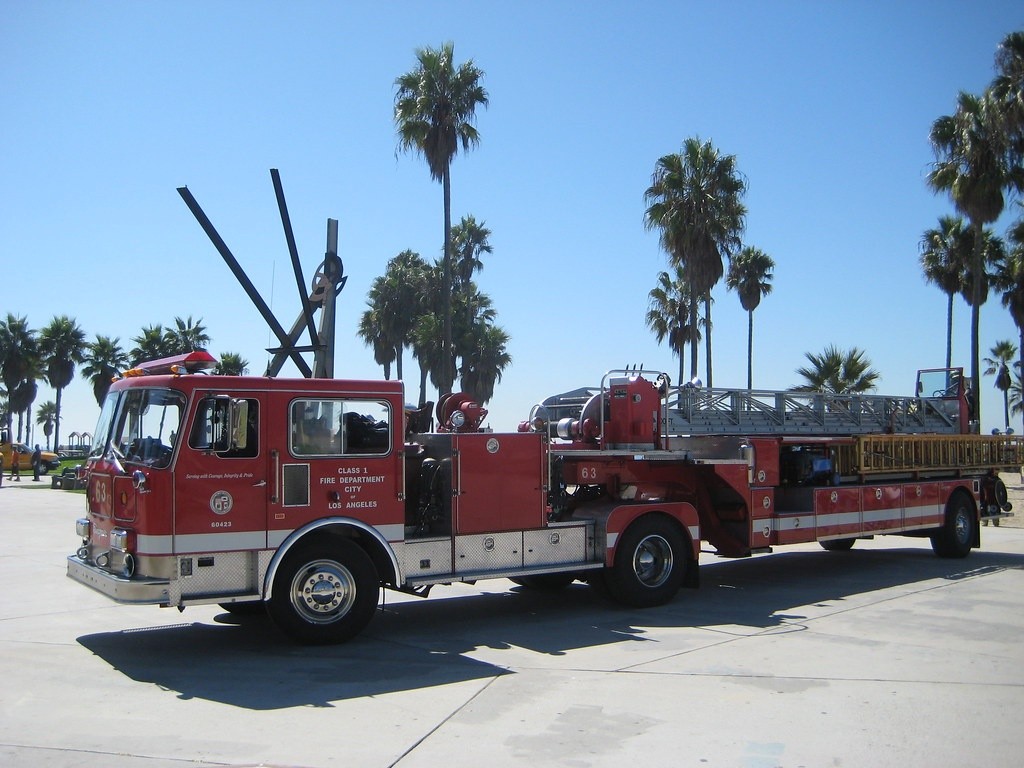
[{"x1": 0, "y1": 443, "x2": 62, "y2": 475}]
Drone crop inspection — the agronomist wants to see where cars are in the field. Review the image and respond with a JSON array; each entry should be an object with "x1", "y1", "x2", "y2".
[{"x1": 59, "y1": 452, "x2": 68, "y2": 459}]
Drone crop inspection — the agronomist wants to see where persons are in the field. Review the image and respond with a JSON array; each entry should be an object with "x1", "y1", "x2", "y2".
[
  {"x1": 31, "y1": 444, "x2": 41, "y2": 481},
  {"x1": 982, "y1": 468, "x2": 1000, "y2": 527},
  {"x1": 210, "y1": 408, "x2": 258, "y2": 457},
  {"x1": 6, "y1": 446, "x2": 20, "y2": 481},
  {"x1": 0, "y1": 443, "x2": 4, "y2": 487}
]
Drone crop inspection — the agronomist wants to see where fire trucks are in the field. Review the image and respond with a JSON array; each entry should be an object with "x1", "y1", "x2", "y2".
[{"x1": 65, "y1": 352, "x2": 1016, "y2": 642}]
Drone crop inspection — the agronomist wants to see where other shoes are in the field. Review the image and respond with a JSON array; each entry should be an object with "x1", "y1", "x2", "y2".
[
  {"x1": 6, "y1": 477, "x2": 11, "y2": 481},
  {"x1": 14, "y1": 478, "x2": 20, "y2": 481},
  {"x1": 32, "y1": 479, "x2": 39, "y2": 481}
]
